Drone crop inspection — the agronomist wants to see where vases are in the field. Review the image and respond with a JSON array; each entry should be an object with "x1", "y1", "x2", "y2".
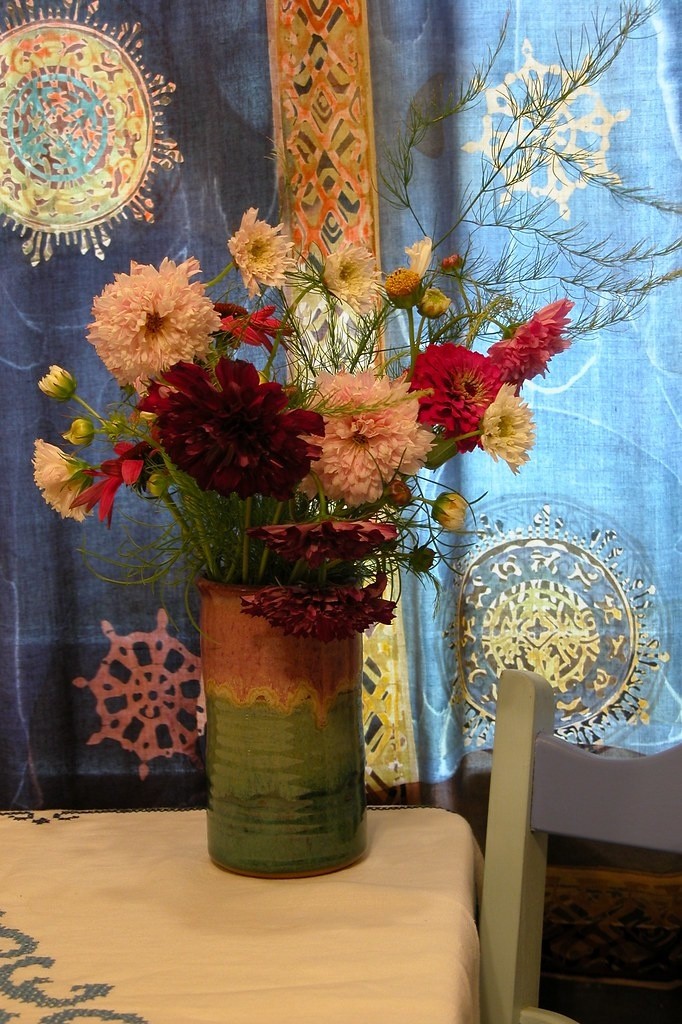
[{"x1": 196, "y1": 576, "x2": 368, "y2": 880}]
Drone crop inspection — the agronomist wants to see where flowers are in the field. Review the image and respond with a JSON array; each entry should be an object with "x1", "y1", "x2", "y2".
[{"x1": 26, "y1": 0, "x2": 681, "y2": 644}]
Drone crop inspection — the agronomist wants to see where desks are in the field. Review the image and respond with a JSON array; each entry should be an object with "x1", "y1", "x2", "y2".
[{"x1": 0, "y1": 806, "x2": 485, "y2": 1024}]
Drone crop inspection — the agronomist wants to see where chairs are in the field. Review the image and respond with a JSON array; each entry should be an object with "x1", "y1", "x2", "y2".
[{"x1": 481, "y1": 670, "x2": 682, "y2": 1024}]
